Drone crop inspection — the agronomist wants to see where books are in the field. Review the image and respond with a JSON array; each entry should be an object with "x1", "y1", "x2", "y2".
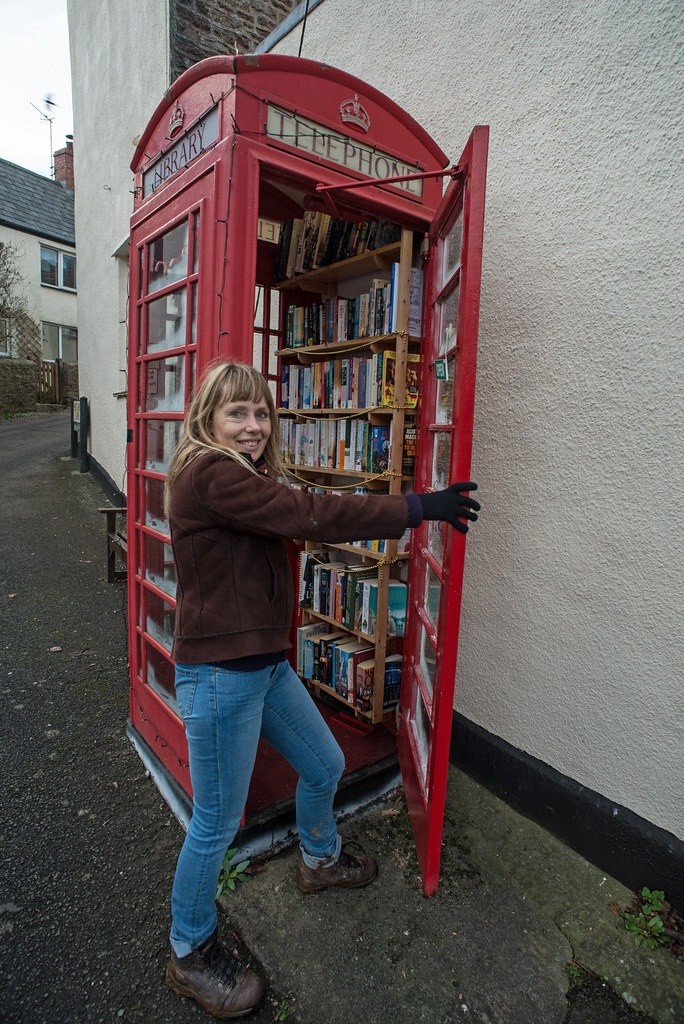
[{"x1": 273, "y1": 209, "x2": 425, "y2": 719}]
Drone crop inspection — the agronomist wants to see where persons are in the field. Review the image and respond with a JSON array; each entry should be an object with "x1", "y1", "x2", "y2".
[{"x1": 170, "y1": 360, "x2": 481, "y2": 1017}]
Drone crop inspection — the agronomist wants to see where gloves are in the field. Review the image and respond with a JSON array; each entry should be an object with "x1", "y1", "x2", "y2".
[{"x1": 418, "y1": 480, "x2": 483, "y2": 534}]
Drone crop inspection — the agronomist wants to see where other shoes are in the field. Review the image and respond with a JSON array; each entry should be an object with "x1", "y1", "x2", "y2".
[
  {"x1": 297, "y1": 841, "x2": 378, "y2": 894},
  {"x1": 165, "y1": 923, "x2": 266, "y2": 1019}
]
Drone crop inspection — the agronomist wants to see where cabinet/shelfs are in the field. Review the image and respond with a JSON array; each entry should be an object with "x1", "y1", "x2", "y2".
[{"x1": 274, "y1": 228, "x2": 413, "y2": 724}]
